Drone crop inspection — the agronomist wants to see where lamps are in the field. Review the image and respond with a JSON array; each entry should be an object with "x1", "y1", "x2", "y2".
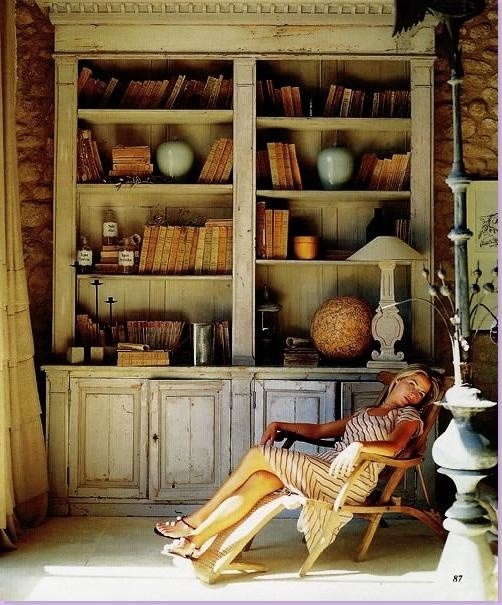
[{"x1": 345, "y1": 235, "x2": 428, "y2": 371}]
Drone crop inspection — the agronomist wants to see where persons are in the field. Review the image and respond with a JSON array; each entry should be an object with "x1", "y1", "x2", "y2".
[{"x1": 153, "y1": 362, "x2": 439, "y2": 562}]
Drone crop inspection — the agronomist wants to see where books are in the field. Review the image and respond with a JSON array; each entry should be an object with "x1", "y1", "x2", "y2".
[
  {"x1": 76, "y1": 314, "x2": 186, "y2": 365},
  {"x1": 325, "y1": 84, "x2": 410, "y2": 118},
  {"x1": 357, "y1": 151, "x2": 410, "y2": 190},
  {"x1": 256, "y1": 80, "x2": 304, "y2": 117},
  {"x1": 77, "y1": 128, "x2": 154, "y2": 183},
  {"x1": 92, "y1": 218, "x2": 233, "y2": 276},
  {"x1": 256, "y1": 201, "x2": 290, "y2": 260},
  {"x1": 216, "y1": 320, "x2": 231, "y2": 361},
  {"x1": 199, "y1": 137, "x2": 234, "y2": 185},
  {"x1": 255, "y1": 142, "x2": 304, "y2": 191},
  {"x1": 79, "y1": 63, "x2": 233, "y2": 108}
]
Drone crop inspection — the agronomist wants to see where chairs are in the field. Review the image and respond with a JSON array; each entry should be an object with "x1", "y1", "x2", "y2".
[{"x1": 173, "y1": 370, "x2": 449, "y2": 585}]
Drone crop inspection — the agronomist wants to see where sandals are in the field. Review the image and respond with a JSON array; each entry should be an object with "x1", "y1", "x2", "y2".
[
  {"x1": 154, "y1": 511, "x2": 196, "y2": 539},
  {"x1": 161, "y1": 536, "x2": 202, "y2": 562}
]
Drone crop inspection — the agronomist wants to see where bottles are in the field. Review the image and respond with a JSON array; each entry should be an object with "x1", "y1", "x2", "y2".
[
  {"x1": 119, "y1": 239, "x2": 135, "y2": 275},
  {"x1": 77, "y1": 240, "x2": 93, "y2": 274},
  {"x1": 294, "y1": 236, "x2": 318, "y2": 259},
  {"x1": 102, "y1": 211, "x2": 119, "y2": 245}
]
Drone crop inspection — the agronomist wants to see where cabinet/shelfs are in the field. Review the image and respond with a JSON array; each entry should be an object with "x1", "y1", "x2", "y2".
[
  {"x1": 52, "y1": 53, "x2": 242, "y2": 365},
  {"x1": 244, "y1": 54, "x2": 438, "y2": 367},
  {"x1": 241, "y1": 368, "x2": 406, "y2": 518},
  {"x1": 41, "y1": 367, "x2": 240, "y2": 518}
]
